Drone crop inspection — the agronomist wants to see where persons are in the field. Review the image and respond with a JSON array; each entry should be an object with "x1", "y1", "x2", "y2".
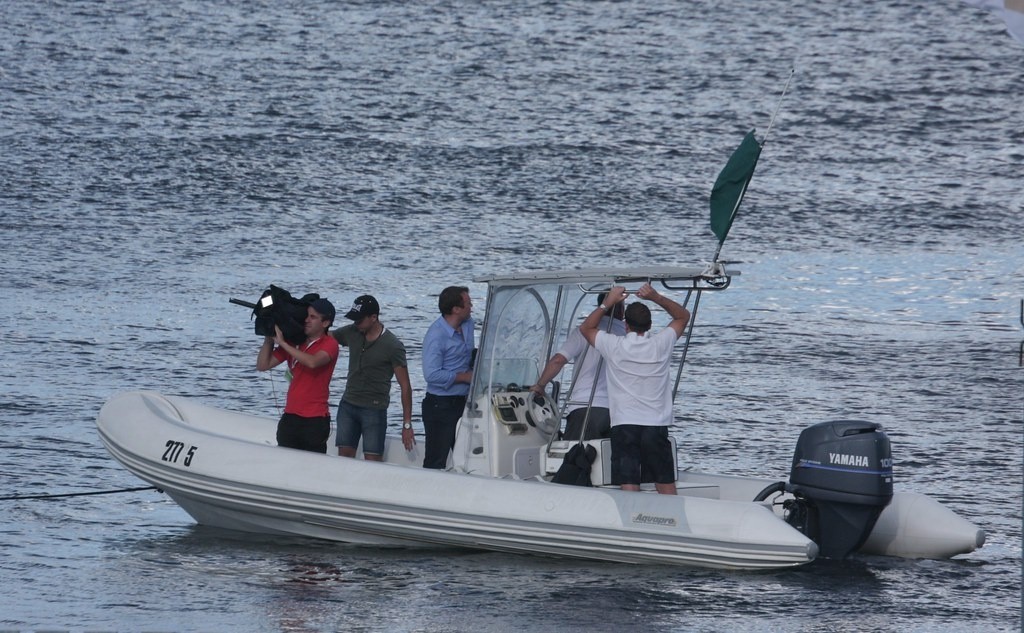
[
  {"x1": 580, "y1": 283, "x2": 690, "y2": 495},
  {"x1": 531, "y1": 288, "x2": 627, "y2": 440},
  {"x1": 421, "y1": 286, "x2": 475, "y2": 469},
  {"x1": 329, "y1": 295, "x2": 416, "y2": 461},
  {"x1": 257, "y1": 298, "x2": 339, "y2": 453}
]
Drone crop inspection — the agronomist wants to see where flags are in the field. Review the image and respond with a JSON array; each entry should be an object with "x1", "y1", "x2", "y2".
[{"x1": 710, "y1": 129, "x2": 761, "y2": 240}]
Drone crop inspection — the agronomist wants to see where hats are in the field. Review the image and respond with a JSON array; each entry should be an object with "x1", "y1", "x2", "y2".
[
  {"x1": 344, "y1": 295, "x2": 379, "y2": 320},
  {"x1": 304, "y1": 298, "x2": 336, "y2": 319}
]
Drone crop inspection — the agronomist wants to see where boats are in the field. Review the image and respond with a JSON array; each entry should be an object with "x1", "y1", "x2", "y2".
[{"x1": 95, "y1": 65, "x2": 987, "y2": 571}]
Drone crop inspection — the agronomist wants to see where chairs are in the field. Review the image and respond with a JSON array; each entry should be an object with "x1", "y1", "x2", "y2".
[{"x1": 539, "y1": 437, "x2": 678, "y2": 487}]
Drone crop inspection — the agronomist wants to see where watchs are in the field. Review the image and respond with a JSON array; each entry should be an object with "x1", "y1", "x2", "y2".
[
  {"x1": 403, "y1": 424, "x2": 412, "y2": 429},
  {"x1": 600, "y1": 304, "x2": 608, "y2": 312}
]
